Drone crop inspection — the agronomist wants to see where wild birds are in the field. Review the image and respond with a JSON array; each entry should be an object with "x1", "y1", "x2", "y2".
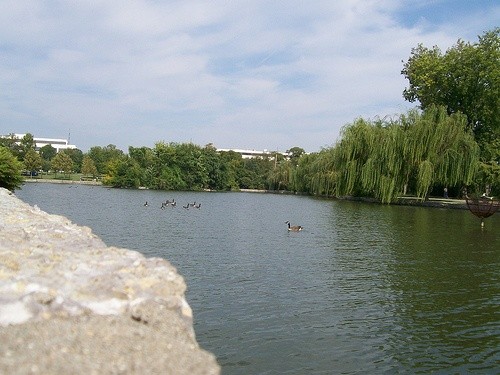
[
  {"x1": 285, "y1": 221, "x2": 303, "y2": 231},
  {"x1": 143, "y1": 199, "x2": 202, "y2": 210}
]
